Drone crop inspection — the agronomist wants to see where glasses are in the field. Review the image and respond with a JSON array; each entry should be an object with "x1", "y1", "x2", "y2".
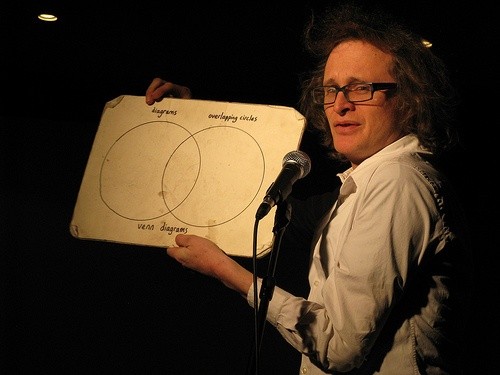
[{"x1": 309, "y1": 81, "x2": 404, "y2": 106}]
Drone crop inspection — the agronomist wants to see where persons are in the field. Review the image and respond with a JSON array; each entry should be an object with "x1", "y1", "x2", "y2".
[{"x1": 146, "y1": 4, "x2": 458, "y2": 375}]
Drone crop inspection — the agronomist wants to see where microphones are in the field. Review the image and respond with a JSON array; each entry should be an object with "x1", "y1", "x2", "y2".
[{"x1": 255, "y1": 150, "x2": 312, "y2": 220}]
